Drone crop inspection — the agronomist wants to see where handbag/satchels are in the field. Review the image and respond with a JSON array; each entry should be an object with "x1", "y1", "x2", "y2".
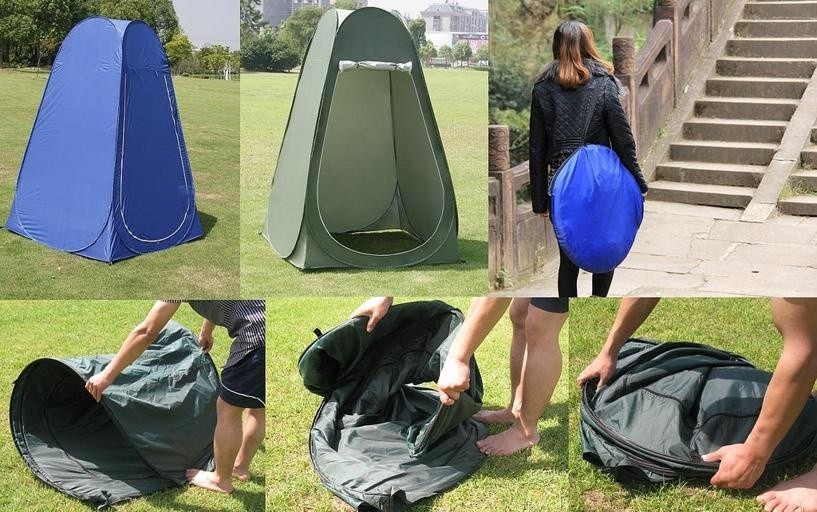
[{"x1": 547, "y1": 74, "x2": 645, "y2": 274}]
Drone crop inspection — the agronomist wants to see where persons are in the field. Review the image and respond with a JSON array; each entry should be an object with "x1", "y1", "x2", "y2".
[
  {"x1": 346, "y1": 296, "x2": 568, "y2": 459},
  {"x1": 527, "y1": 23, "x2": 649, "y2": 298},
  {"x1": 575, "y1": 297, "x2": 817, "y2": 512},
  {"x1": 84, "y1": 300, "x2": 265, "y2": 493}
]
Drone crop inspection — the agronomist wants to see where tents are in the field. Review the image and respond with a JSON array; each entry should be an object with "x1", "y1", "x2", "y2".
[
  {"x1": 5, "y1": 16, "x2": 204, "y2": 265},
  {"x1": 258, "y1": 7, "x2": 464, "y2": 272}
]
